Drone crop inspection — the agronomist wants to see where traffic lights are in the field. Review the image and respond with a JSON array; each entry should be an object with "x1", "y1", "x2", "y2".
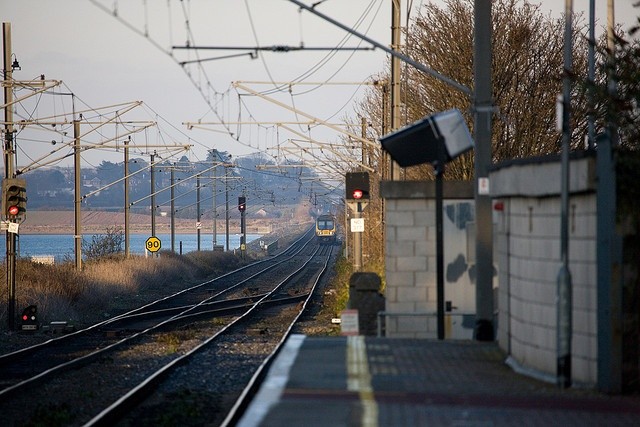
[
  {"x1": 2, "y1": 178, "x2": 27, "y2": 226},
  {"x1": 20, "y1": 305, "x2": 39, "y2": 334},
  {"x1": 238, "y1": 197, "x2": 246, "y2": 210},
  {"x1": 346, "y1": 172, "x2": 369, "y2": 203}
]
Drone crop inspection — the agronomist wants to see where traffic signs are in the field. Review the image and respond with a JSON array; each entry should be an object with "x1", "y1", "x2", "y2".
[{"x1": 145, "y1": 237, "x2": 161, "y2": 252}]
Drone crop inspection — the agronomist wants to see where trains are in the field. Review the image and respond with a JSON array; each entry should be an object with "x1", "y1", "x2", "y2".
[{"x1": 316, "y1": 212, "x2": 336, "y2": 244}]
